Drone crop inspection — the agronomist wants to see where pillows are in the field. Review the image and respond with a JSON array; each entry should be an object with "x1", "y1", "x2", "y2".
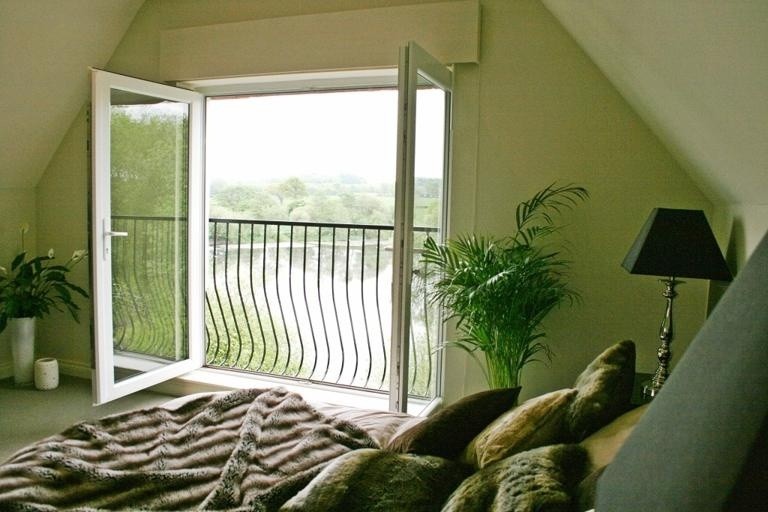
[
  {"x1": 442, "y1": 445, "x2": 587, "y2": 511},
  {"x1": 457, "y1": 389, "x2": 577, "y2": 473},
  {"x1": 279, "y1": 448, "x2": 456, "y2": 512},
  {"x1": 552, "y1": 338, "x2": 636, "y2": 444},
  {"x1": 387, "y1": 386, "x2": 521, "y2": 454}
]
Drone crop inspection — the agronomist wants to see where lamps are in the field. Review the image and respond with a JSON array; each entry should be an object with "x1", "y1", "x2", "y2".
[{"x1": 621, "y1": 207, "x2": 732, "y2": 397}]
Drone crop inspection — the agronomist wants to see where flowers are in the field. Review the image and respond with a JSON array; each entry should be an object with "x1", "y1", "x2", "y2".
[{"x1": 0, "y1": 222, "x2": 91, "y2": 335}]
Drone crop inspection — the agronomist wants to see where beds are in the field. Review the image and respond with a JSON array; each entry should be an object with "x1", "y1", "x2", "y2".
[{"x1": 0, "y1": 387, "x2": 417, "y2": 511}]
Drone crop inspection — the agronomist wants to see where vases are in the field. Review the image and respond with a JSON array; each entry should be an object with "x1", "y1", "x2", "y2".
[{"x1": 5, "y1": 316, "x2": 59, "y2": 390}]
